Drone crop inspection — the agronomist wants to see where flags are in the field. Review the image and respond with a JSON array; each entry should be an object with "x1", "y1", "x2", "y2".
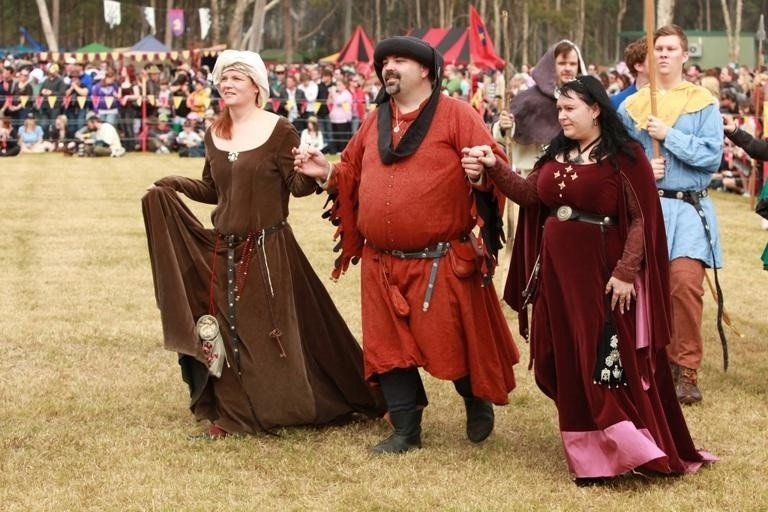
[
  {"x1": 467, "y1": 1, "x2": 506, "y2": 73},
  {"x1": 103, "y1": 0, "x2": 123, "y2": 29},
  {"x1": 167, "y1": 9, "x2": 186, "y2": 36},
  {"x1": 198, "y1": 8, "x2": 212, "y2": 40},
  {"x1": 143, "y1": 7, "x2": 157, "y2": 36}
]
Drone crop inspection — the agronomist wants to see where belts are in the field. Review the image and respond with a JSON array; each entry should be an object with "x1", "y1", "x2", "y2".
[
  {"x1": 365, "y1": 239, "x2": 450, "y2": 312},
  {"x1": 654, "y1": 187, "x2": 730, "y2": 373},
  {"x1": 546, "y1": 205, "x2": 615, "y2": 226},
  {"x1": 214, "y1": 221, "x2": 286, "y2": 375}
]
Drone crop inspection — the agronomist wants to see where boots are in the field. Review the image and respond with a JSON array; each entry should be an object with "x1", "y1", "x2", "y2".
[
  {"x1": 455, "y1": 378, "x2": 495, "y2": 442},
  {"x1": 372, "y1": 406, "x2": 421, "y2": 455},
  {"x1": 678, "y1": 364, "x2": 702, "y2": 403}
]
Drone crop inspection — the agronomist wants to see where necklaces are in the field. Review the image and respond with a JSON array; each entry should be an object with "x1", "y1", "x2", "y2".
[
  {"x1": 571, "y1": 133, "x2": 603, "y2": 165},
  {"x1": 393, "y1": 96, "x2": 428, "y2": 134}
]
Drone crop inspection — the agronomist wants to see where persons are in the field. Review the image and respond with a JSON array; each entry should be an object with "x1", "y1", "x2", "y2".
[
  {"x1": 719, "y1": 92, "x2": 740, "y2": 115},
  {"x1": 30, "y1": 52, "x2": 50, "y2": 83},
  {"x1": 93, "y1": 60, "x2": 110, "y2": 83},
  {"x1": 8, "y1": 67, "x2": 33, "y2": 131},
  {"x1": 0, "y1": 116, "x2": 21, "y2": 157},
  {"x1": 186, "y1": 78, "x2": 214, "y2": 122},
  {"x1": 0, "y1": 66, "x2": 17, "y2": 118},
  {"x1": 492, "y1": 61, "x2": 506, "y2": 114},
  {"x1": 269, "y1": 63, "x2": 288, "y2": 114},
  {"x1": 686, "y1": 62, "x2": 707, "y2": 84},
  {"x1": 74, "y1": 110, "x2": 97, "y2": 142},
  {"x1": 147, "y1": 113, "x2": 177, "y2": 155},
  {"x1": 443, "y1": 63, "x2": 462, "y2": 96},
  {"x1": 90, "y1": 69, "x2": 119, "y2": 127},
  {"x1": 18, "y1": 112, "x2": 44, "y2": 153},
  {"x1": 196, "y1": 67, "x2": 211, "y2": 88},
  {"x1": 508, "y1": 63, "x2": 530, "y2": 103},
  {"x1": 616, "y1": 74, "x2": 631, "y2": 90},
  {"x1": 327, "y1": 79, "x2": 353, "y2": 155},
  {"x1": 599, "y1": 72, "x2": 609, "y2": 90},
  {"x1": 605, "y1": 70, "x2": 621, "y2": 97},
  {"x1": 469, "y1": 74, "x2": 719, "y2": 481},
  {"x1": 39, "y1": 63, "x2": 68, "y2": 139},
  {"x1": 44, "y1": 114, "x2": 76, "y2": 152},
  {"x1": 170, "y1": 73, "x2": 193, "y2": 118},
  {"x1": 74, "y1": 62, "x2": 94, "y2": 157},
  {"x1": 457, "y1": 68, "x2": 470, "y2": 95},
  {"x1": 588, "y1": 64, "x2": 602, "y2": 82},
  {"x1": 720, "y1": 154, "x2": 744, "y2": 194},
  {"x1": 720, "y1": 113, "x2": 768, "y2": 271},
  {"x1": 617, "y1": 23, "x2": 745, "y2": 404},
  {"x1": 718, "y1": 66, "x2": 744, "y2": 102},
  {"x1": 139, "y1": 48, "x2": 388, "y2": 438},
  {"x1": 737, "y1": 99, "x2": 762, "y2": 139},
  {"x1": 292, "y1": 35, "x2": 522, "y2": 452},
  {"x1": 117, "y1": 64, "x2": 140, "y2": 151},
  {"x1": 279, "y1": 75, "x2": 308, "y2": 131},
  {"x1": 607, "y1": 34, "x2": 650, "y2": 111},
  {"x1": 179, "y1": 112, "x2": 206, "y2": 158},
  {"x1": 146, "y1": 64, "x2": 161, "y2": 98},
  {"x1": 346, "y1": 73, "x2": 358, "y2": 97},
  {"x1": 489, "y1": 37, "x2": 589, "y2": 256},
  {"x1": 701, "y1": 75, "x2": 719, "y2": 106},
  {"x1": 736, "y1": 71, "x2": 754, "y2": 98},
  {"x1": 316, "y1": 68, "x2": 337, "y2": 155},
  {"x1": 732, "y1": 115, "x2": 754, "y2": 198},
  {"x1": 327, "y1": 67, "x2": 345, "y2": 113},
  {"x1": 83, "y1": 116, "x2": 126, "y2": 157},
  {"x1": 62, "y1": 66, "x2": 82, "y2": 150},
  {"x1": 452, "y1": 88, "x2": 463, "y2": 98},
  {"x1": 297, "y1": 73, "x2": 319, "y2": 131},
  {"x1": 154, "y1": 80, "x2": 177, "y2": 121},
  {"x1": 300, "y1": 115, "x2": 328, "y2": 154},
  {"x1": 352, "y1": 74, "x2": 368, "y2": 135},
  {"x1": 178, "y1": 121, "x2": 202, "y2": 147}
]
